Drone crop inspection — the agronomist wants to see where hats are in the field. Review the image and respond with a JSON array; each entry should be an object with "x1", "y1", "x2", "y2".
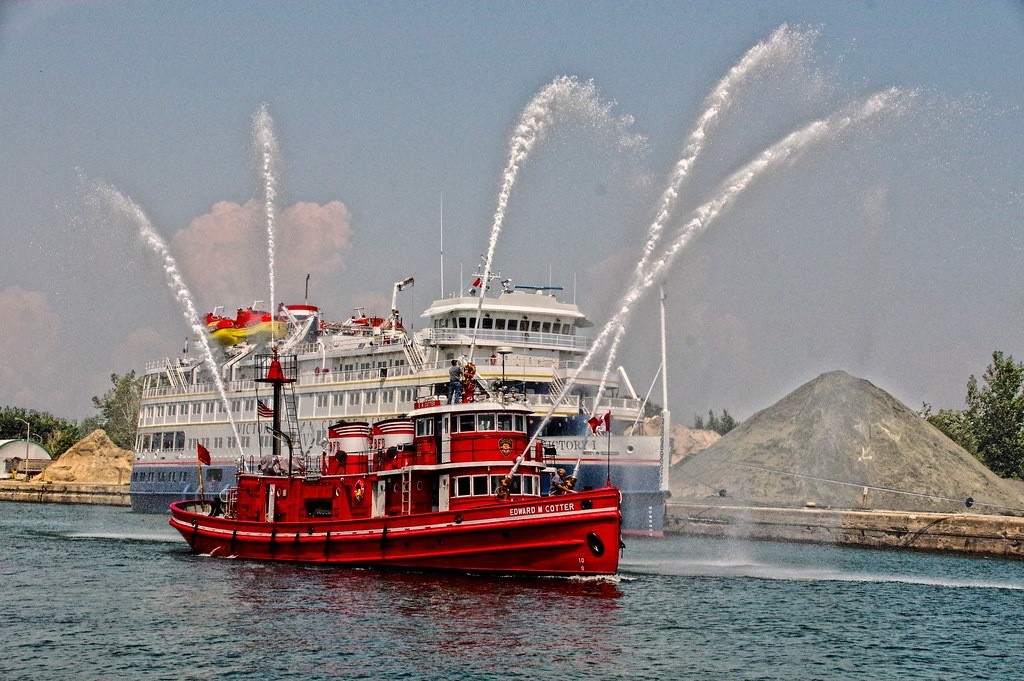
[{"x1": 451, "y1": 360, "x2": 457, "y2": 364}]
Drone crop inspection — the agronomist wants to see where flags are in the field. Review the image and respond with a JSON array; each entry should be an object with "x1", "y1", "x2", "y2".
[
  {"x1": 587, "y1": 411, "x2": 610, "y2": 433},
  {"x1": 257, "y1": 398, "x2": 273, "y2": 417},
  {"x1": 198, "y1": 443, "x2": 212, "y2": 466}
]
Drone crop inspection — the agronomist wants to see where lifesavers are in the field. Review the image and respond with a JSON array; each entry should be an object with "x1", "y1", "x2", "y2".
[
  {"x1": 353, "y1": 479, "x2": 364, "y2": 500},
  {"x1": 463, "y1": 364, "x2": 475, "y2": 378},
  {"x1": 314, "y1": 367, "x2": 320, "y2": 375},
  {"x1": 490, "y1": 354, "x2": 496, "y2": 366}
]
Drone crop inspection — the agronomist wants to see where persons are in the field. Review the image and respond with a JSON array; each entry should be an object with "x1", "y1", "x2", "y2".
[
  {"x1": 447, "y1": 359, "x2": 464, "y2": 404},
  {"x1": 549, "y1": 468, "x2": 577, "y2": 496}
]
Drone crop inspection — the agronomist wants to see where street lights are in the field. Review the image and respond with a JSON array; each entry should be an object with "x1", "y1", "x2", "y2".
[
  {"x1": 33, "y1": 433, "x2": 42, "y2": 444},
  {"x1": 15, "y1": 417, "x2": 29, "y2": 482}
]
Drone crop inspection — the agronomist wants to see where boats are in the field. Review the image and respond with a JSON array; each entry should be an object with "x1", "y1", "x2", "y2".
[
  {"x1": 169, "y1": 343, "x2": 626, "y2": 576},
  {"x1": 130, "y1": 195, "x2": 670, "y2": 538}
]
